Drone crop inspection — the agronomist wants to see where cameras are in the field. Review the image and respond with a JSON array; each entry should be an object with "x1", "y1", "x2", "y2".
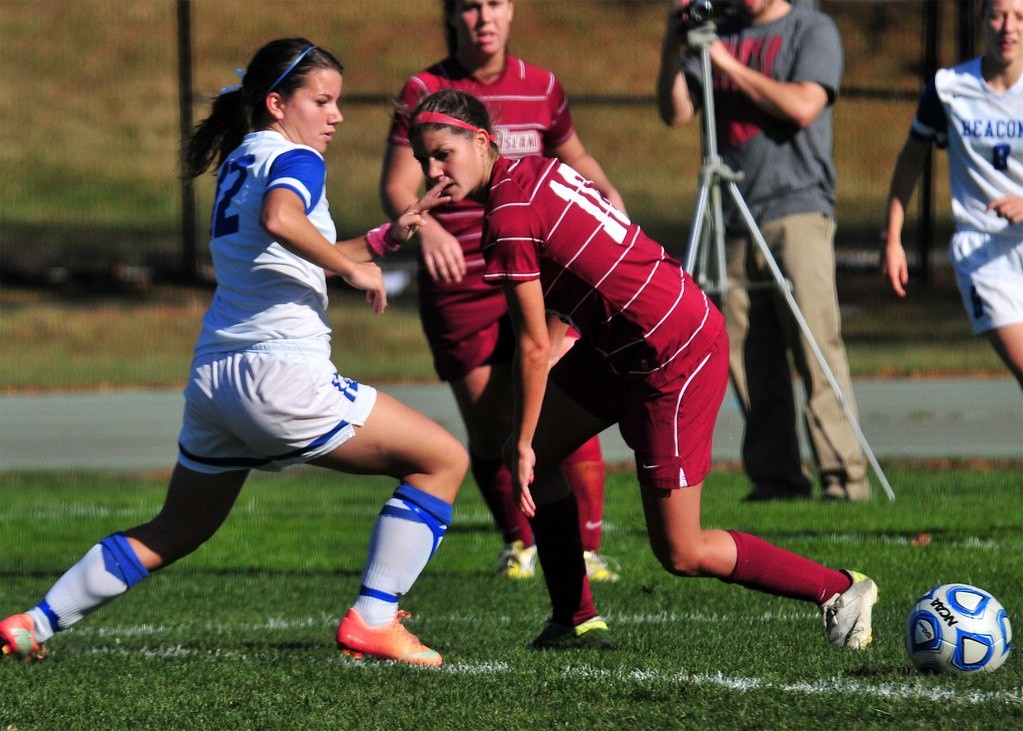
[{"x1": 674, "y1": 0, "x2": 746, "y2": 42}]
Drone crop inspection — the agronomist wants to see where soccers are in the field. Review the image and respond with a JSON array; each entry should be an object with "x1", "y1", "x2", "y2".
[{"x1": 906, "y1": 584, "x2": 1013, "y2": 676}]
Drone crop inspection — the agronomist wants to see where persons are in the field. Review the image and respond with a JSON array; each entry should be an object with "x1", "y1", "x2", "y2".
[
  {"x1": 0, "y1": 35, "x2": 471, "y2": 669},
  {"x1": 650, "y1": 0, "x2": 873, "y2": 498},
  {"x1": 379, "y1": 0, "x2": 624, "y2": 588},
  {"x1": 886, "y1": 1, "x2": 1023, "y2": 388},
  {"x1": 408, "y1": 87, "x2": 879, "y2": 658}
]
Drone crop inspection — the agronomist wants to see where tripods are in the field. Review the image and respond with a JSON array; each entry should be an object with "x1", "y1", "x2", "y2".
[{"x1": 655, "y1": 44, "x2": 898, "y2": 506}]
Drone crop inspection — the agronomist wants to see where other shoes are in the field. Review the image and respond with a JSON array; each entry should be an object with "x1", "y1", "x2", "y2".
[{"x1": 738, "y1": 482, "x2": 812, "y2": 499}]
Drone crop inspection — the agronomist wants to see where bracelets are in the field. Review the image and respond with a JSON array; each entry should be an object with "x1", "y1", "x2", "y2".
[{"x1": 364, "y1": 221, "x2": 400, "y2": 257}]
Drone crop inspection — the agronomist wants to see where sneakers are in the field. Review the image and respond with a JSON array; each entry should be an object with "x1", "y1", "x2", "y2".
[
  {"x1": 823, "y1": 570, "x2": 880, "y2": 651},
  {"x1": 336, "y1": 607, "x2": 444, "y2": 670},
  {"x1": 0, "y1": 615, "x2": 46, "y2": 661},
  {"x1": 580, "y1": 550, "x2": 620, "y2": 583},
  {"x1": 526, "y1": 615, "x2": 614, "y2": 651},
  {"x1": 496, "y1": 541, "x2": 538, "y2": 578}
]
